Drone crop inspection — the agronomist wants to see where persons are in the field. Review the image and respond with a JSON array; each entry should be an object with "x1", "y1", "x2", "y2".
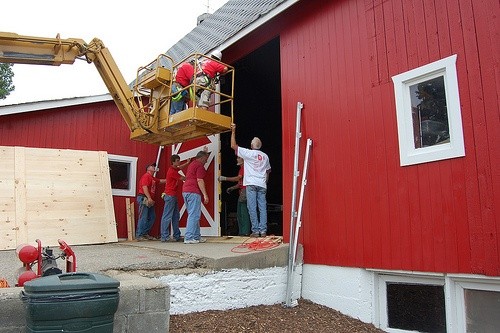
[
  {"x1": 170, "y1": 59, "x2": 197, "y2": 115},
  {"x1": 182, "y1": 150, "x2": 209, "y2": 244},
  {"x1": 230, "y1": 122, "x2": 271, "y2": 237},
  {"x1": 161, "y1": 155, "x2": 185, "y2": 242},
  {"x1": 136, "y1": 165, "x2": 166, "y2": 242},
  {"x1": 218, "y1": 157, "x2": 251, "y2": 236},
  {"x1": 193, "y1": 50, "x2": 222, "y2": 110}
]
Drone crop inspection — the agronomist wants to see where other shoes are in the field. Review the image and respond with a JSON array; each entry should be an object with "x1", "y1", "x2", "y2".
[
  {"x1": 250, "y1": 232, "x2": 266, "y2": 238},
  {"x1": 183, "y1": 238, "x2": 206, "y2": 244},
  {"x1": 135, "y1": 235, "x2": 157, "y2": 241},
  {"x1": 172, "y1": 237, "x2": 184, "y2": 242},
  {"x1": 161, "y1": 238, "x2": 177, "y2": 242}
]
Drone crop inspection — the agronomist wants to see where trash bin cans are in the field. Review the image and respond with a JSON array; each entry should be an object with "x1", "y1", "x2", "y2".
[{"x1": 20, "y1": 270, "x2": 122, "y2": 333}]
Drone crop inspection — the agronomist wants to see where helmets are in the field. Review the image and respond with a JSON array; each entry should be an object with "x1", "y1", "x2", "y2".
[
  {"x1": 211, "y1": 50, "x2": 222, "y2": 60},
  {"x1": 191, "y1": 58, "x2": 199, "y2": 65}
]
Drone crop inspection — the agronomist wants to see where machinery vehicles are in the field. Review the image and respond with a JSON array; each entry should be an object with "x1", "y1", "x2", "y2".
[{"x1": 0, "y1": 31, "x2": 236, "y2": 146}]
